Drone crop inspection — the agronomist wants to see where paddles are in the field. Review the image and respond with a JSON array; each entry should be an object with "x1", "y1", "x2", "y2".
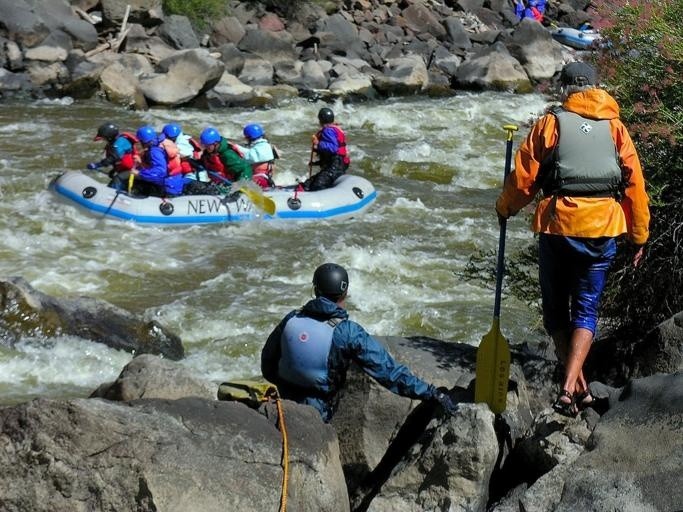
[
  {"x1": 189, "y1": 159, "x2": 276, "y2": 216},
  {"x1": 475, "y1": 124, "x2": 517, "y2": 413},
  {"x1": 125, "y1": 156, "x2": 139, "y2": 197}
]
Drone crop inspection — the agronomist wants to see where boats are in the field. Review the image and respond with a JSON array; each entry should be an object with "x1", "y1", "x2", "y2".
[
  {"x1": 46, "y1": 167, "x2": 378, "y2": 227},
  {"x1": 551, "y1": 27, "x2": 625, "y2": 49}
]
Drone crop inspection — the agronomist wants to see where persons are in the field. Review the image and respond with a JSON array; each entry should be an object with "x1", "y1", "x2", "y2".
[
  {"x1": 261, "y1": 263, "x2": 459, "y2": 422},
  {"x1": 305, "y1": 107, "x2": 350, "y2": 192},
  {"x1": 88, "y1": 123, "x2": 277, "y2": 195},
  {"x1": 496, "y1": 61, "x2": 651, "y2": 417}
]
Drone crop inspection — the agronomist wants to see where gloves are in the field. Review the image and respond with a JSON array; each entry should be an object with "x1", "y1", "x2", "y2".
[{"x1": 433, "y1": 391, "x2": 456, "y2": 413}]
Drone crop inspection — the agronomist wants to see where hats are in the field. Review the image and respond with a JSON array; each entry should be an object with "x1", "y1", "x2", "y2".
[{"x1": 561, "y1": 62, "x2": 597, "y2": 86}]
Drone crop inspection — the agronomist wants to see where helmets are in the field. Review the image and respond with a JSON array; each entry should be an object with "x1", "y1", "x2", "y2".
[
  {"x1": 319, "y1": 108, "x2": 334, "y2": 123},
  {"x1": 244, "y1": 124, "x2": 262, "y2": 140},
  {"x1": 98, "y1": 123, "x2": 118, "y2": 139},
  {"x1": 200, "y1": 128, "x2": 221, "y2": 145},
  {"x1": 312, "y1": 263, "x2": 348, "y2": 295},
  {"x1": 134, "y1": 124, "x2": 180, "y2": 143}
]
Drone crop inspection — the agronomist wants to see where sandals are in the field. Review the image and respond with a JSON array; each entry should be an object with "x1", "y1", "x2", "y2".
[{"x1": 554, "y1": 389, "x2": 595, "y2": 417}]
